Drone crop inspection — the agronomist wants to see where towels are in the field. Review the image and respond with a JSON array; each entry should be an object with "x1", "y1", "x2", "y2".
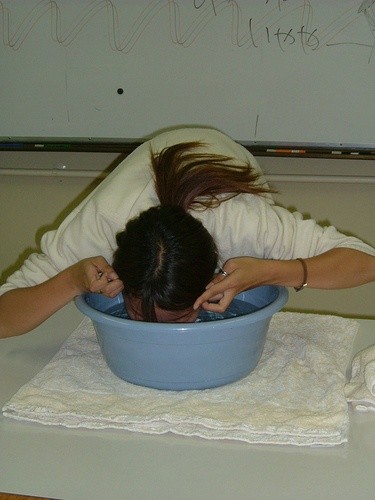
[
  {"x1": 0, "y1": 309, "x2": 360, "y2": 449},
  {"x1": 345, "y1": 343, "x2": 375, "y2": 412}
]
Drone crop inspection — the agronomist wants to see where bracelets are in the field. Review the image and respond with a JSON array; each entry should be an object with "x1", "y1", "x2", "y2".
[{"x1": 293, "y1": 258, "x2": 307, "y2": 292}]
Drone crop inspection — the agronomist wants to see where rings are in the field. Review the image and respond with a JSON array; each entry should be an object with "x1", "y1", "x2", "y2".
[
  {"x1": 219, "y1": 269, "x2": 229, "y2": 277},
  {"x1": 98, "y1": 289, "x2": 103, "y2": 294}
]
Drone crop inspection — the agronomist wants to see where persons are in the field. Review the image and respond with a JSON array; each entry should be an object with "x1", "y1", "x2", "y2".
[{"x1": 0, "y1": 127, "x2": 375, "y2": 349}]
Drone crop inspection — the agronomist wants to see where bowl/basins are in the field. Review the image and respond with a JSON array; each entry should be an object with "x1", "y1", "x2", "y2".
[{"x1": 72, "y1": 281, "x2": 290, "y2": 391}]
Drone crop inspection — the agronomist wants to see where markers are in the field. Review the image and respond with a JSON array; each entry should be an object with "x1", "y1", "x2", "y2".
[{"x1": 266, "y1": 147, "x2": 308, "y2": 154}]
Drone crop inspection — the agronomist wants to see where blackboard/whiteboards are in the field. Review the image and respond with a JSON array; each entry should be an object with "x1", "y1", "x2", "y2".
[{"x1": 0, "y1": 0, "x2": 375, "y2": 161}]
